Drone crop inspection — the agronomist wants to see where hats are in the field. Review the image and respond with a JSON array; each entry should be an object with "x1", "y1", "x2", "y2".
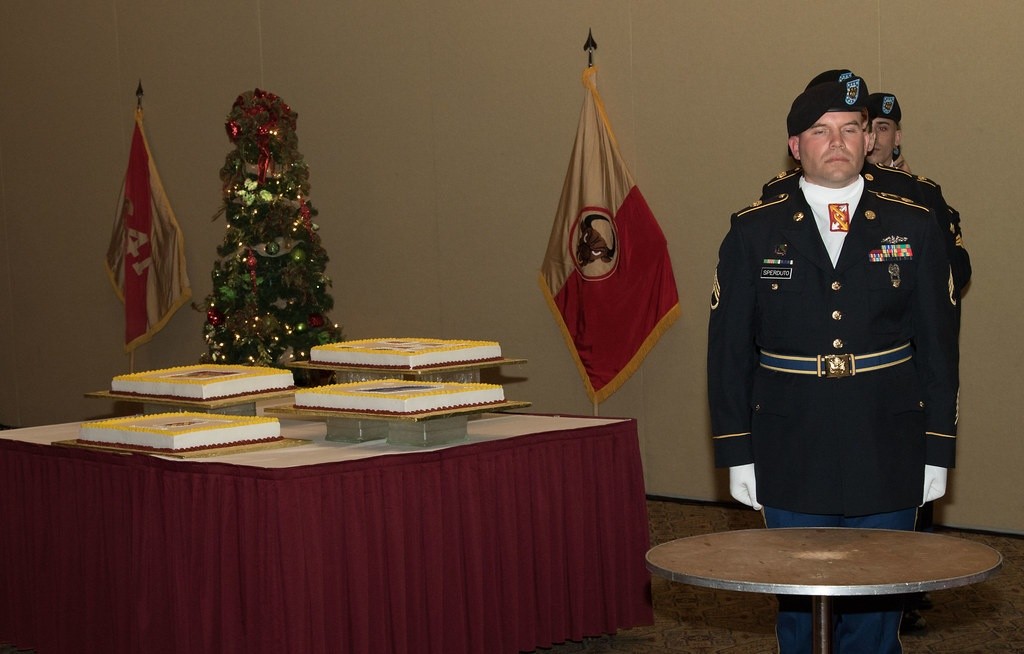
[
  {"x1": 804, "y1": 69, "x2": 856, "y2": 89},
  {"x1": 786, "y1": 77, "x2": 874, "y2": 134},
  {"x1": 868, "y1": 92, "x2": 902, "y2": 123}
]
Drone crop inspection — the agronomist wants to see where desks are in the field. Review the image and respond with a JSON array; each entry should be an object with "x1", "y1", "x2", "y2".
[
  {"x1": 0, "y1": 414, "x2": 657, "y2": 654},
  {"x1": 644, "y1": 527, "x2": 1005, "y2": 654}
]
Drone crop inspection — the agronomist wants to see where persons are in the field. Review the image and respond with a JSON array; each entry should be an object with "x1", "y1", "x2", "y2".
[{"x1": 707, "y1": 70, "x2": 973, "y2": 654}]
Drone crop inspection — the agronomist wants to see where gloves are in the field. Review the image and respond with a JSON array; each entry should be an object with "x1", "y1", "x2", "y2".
[
  {"x1": 919, "y1": 466, "x2": 947, "y2": 507},
  {"x1": 729, "y1": 463, "x2": 762, "y2": 510}
]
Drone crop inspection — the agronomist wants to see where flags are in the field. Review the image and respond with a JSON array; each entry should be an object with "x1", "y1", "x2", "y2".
[
  {"x1": 104, "y1": 109, "x2": 192, "y2": 355},
  {"x1": 539, "y1": 67, "x2": 680, "y2": 405}
]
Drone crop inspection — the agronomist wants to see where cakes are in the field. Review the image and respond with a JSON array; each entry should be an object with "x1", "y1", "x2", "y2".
[
  {"x1": 109, "y1": 363, "x2": 296, "y2": 401},
  {"x1": 77, "y1": 410, "x2": 284, "y2": 453},
  {"x1": 309, "y1": 338, "x2": 504, "y2": 369},
  {"x1": 293, "y1": 378, "x2": 506, "y2": 415}
]
